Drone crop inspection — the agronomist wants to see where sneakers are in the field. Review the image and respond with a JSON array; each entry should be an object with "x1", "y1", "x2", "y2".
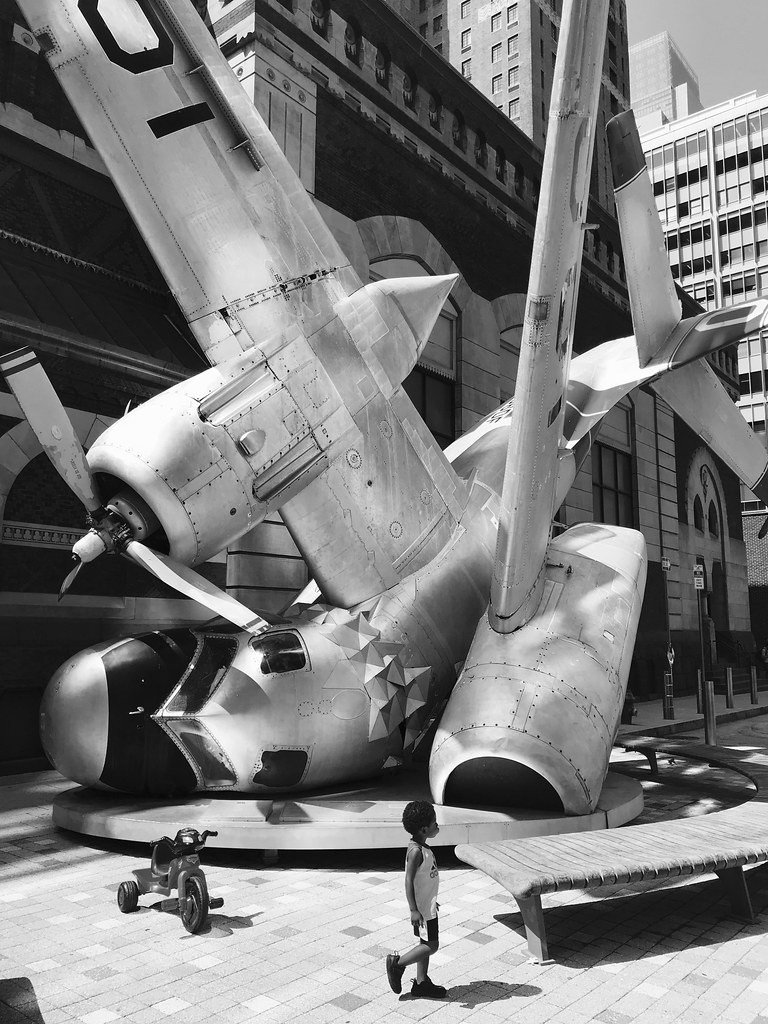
[
  {"x1": 385, "y1": 950, "x2": 406, "y2": 994},
  {"x1": 410, "y1": 975, "x2": 446, "y2": 998}
]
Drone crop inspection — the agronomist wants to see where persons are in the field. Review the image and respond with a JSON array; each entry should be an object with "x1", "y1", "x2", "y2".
[
  {"x1": 386, "y1": 800, "x2": 447, "y2": 998},
  {"x1": 759, "y1": 645, "x2": 768, "y2": 680}
]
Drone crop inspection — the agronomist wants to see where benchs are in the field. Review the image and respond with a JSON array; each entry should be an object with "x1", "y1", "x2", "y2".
[{"x1": 453, "y1": 733, "x2": 768, "y2": 967}]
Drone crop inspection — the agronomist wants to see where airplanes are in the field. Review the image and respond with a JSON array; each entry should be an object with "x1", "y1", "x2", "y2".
[{"x1": 1, "y1": 0, "x2": 764, "y2": 818}]
